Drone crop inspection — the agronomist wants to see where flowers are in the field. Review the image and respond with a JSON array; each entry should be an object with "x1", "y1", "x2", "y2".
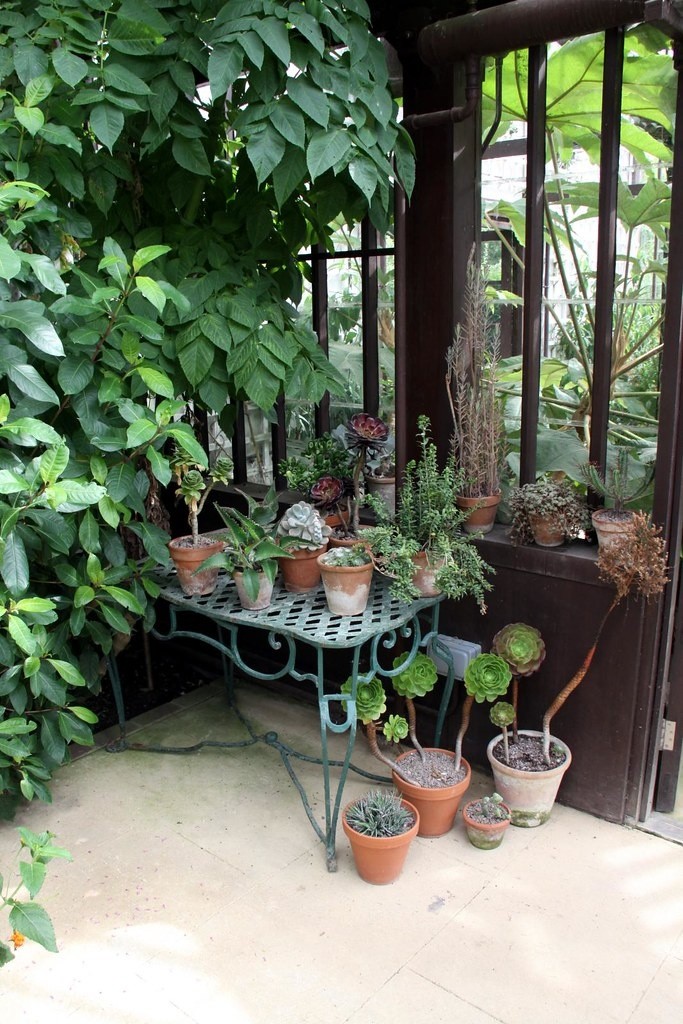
[{"x1": 311, "y1": 413, "x2": 389, "y2": 537}]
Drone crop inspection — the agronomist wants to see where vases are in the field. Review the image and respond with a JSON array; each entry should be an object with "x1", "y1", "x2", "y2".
[{"x1": 329, "y1": 525, "x2": 376, "y2": 548}]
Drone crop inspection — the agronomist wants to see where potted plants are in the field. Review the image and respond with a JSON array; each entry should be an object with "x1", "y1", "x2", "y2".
[
  {"x1": 357, "y1": 416, "x2": 494, "y2": 614},
  {"x1": 166, "y1": 441, "x2": 234, "y2": 595},
  {"x1": 192, "y1": 485, "x2": 318, "y2": 610},
  {"x1": 273, "y1": 501, "x2": 331, "y2": 594},
  {"x1": 507, "y1": 474, "x2": 584, "y2": 549},
  {"x1": 489, "y1": 510, "x2": 670, "y2": 828},
  {"x1": 342, "y1": 787, "x2": 420, "y2": 885},
  {"x1": 342, "y1": 650, "x2": 511, "y2": 837},
  {"x1": 446, "y1": 242, "x2": 502, "y2": 535},
  {"x1": 276, "y1": 430, "x2": 357, "y2": 529},
  {"x1": 317, "y1": 546, "x2": 374, "y2": 615},
  {"x1": 463, "y1": 793, "x2": 513, "y2": 850},
  {"x1": 580, "y1": 448, "x2": 654, "y2": 553},
  {"x1": 366, "y1": 451, "x2": 396, "y2": 517}
]
